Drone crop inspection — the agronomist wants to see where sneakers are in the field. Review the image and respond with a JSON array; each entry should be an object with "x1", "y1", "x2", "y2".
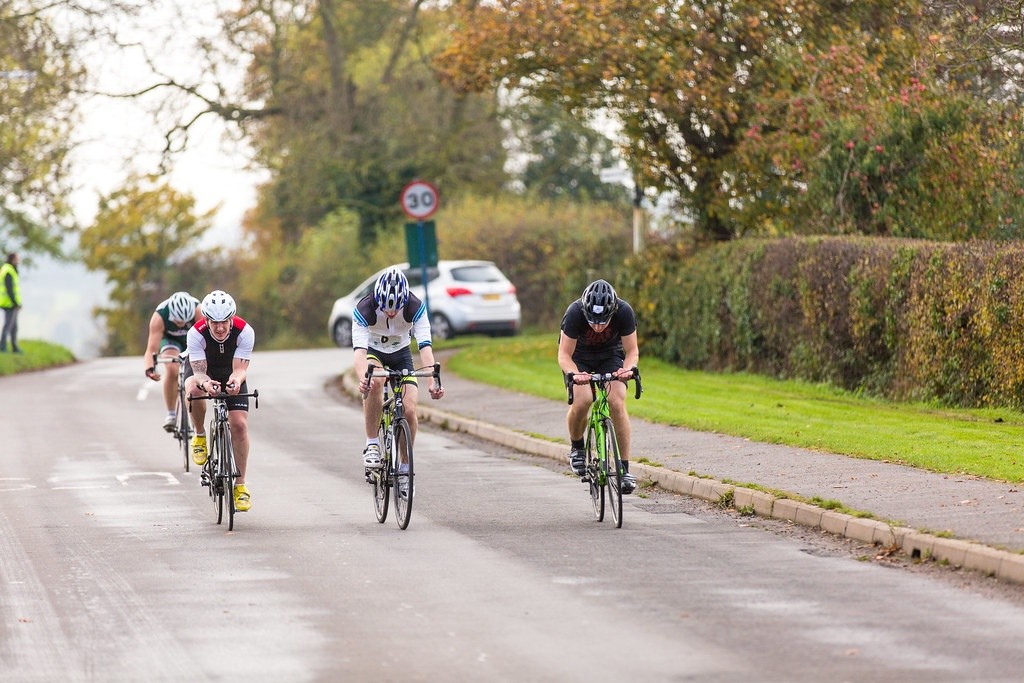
[
  {"x1": 362, "y1": 442, "x2": 381, "y2": 467},
  {"x1": 233, "y1": 485, "x2": 250, "y2": 510},
  {"x1": 398, "y1": 475, "x2": 415, "y2": 501},
  {"x1": 569, "y1": 446, "x2": 586, "y2": 475},
  {"x1": 192, "y1": 436, "x2": 208, "y2": 465},
  {"x1": 620, "y1": 474, "x2": 636, "y2": 491},
  {"x1": 164, "y1": 415, "x2": 176, "y2": 429}
]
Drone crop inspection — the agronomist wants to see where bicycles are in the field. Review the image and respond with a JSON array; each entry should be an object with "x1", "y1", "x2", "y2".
[
  {"x1": 364, "y1": 360, "x2": 444, "y2": 532},
  {"x1": 151, "y1": 351, "x2": 195, "y2": 472},
  {"x1": 567, "y1": 366, "x2": 644, "y2": 529},
  {"x1": 186, "y1": 383, "x2": 258, "y2": 531}
]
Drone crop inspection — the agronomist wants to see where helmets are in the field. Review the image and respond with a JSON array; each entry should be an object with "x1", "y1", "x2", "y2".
[
  {"x1": 167, "y1": 292, "x2": 195, "y2": 321},
  {"x1": 581, "y1": 280, "x2": 618, "y2": 322},
  {"x1": 373, "y1": 268, "x2": 409, "y2": 311},
  {"x1": 201, "y1": 290, "x2": 237, "y2": 322}
]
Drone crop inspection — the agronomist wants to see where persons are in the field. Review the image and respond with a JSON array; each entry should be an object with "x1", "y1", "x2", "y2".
[
  {"x1": 183, "y1": 290, "x2": 256, "y2": 512},
  {"x1": 352, "y1": 268, "x2": 444, "y2": 502},
  {"x1": 0, "y1": 252, "x2": 24, "y2": 354},
  {"x1": 557, "y1": 279, "x2": 639, "y2": 495},
  {"x1": 144, "y1": 291, "x2": 204, "y2": 429}
]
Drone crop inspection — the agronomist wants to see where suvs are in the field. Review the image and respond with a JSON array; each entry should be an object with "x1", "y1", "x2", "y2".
[{"x1": 327, "y1": 259, "x2": 522, "y2": 349}]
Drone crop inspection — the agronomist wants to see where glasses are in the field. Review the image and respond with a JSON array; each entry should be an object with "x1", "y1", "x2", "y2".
[{"x1": 588, "y1": 319, "x2": 610, "y2": 324}]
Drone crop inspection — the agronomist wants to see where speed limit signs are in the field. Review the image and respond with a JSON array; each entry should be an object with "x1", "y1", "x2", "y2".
[{"x1": 401, "y1": 181, "x2": 439, "y2": 220}]
